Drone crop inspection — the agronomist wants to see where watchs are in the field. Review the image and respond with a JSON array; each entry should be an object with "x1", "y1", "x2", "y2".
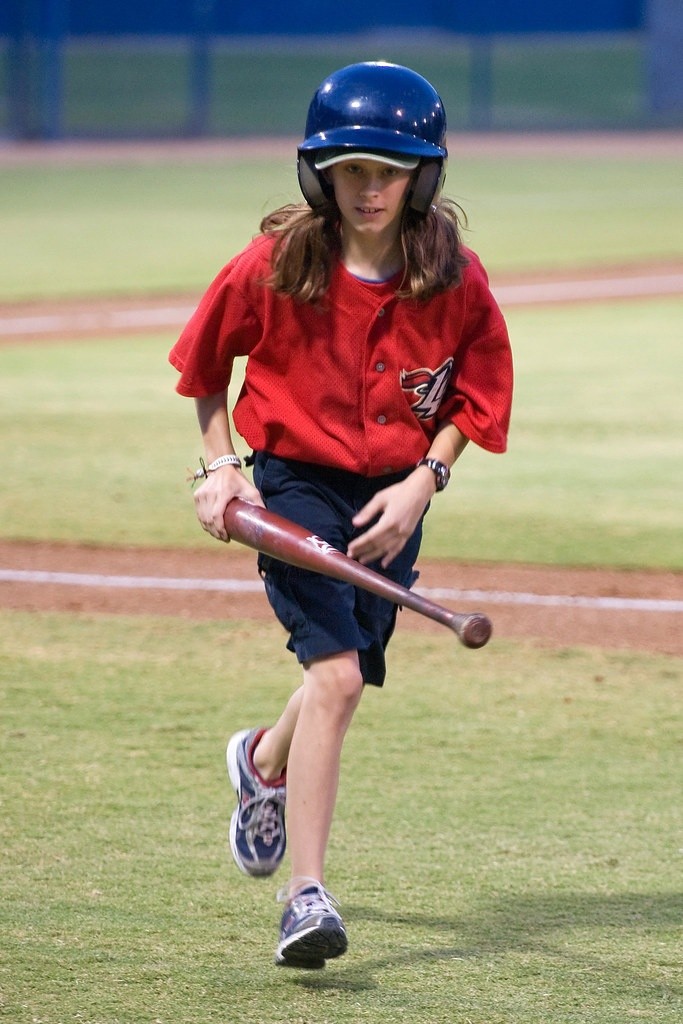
[{"x1": 420, "y1": 459, "x2": 450, "y2": 492}]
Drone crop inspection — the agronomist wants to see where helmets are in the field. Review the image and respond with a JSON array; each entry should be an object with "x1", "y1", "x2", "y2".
[{"x1": 297, "y1": 62, "x2": 448, "y2": 217}]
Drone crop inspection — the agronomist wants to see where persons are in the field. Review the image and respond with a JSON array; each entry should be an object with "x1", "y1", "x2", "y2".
[{"x1": 169, "y1": 61, "x2": 514, "y2": 969}]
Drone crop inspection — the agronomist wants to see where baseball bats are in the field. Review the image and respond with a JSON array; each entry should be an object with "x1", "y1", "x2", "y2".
[{"x1": 224, "y1": 498, "x2": 494, "y2": 650}]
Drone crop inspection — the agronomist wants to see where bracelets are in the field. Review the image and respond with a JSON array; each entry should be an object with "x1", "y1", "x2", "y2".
[{"x1": 195, "y1": 454, "x2": 242, "y2": 480}]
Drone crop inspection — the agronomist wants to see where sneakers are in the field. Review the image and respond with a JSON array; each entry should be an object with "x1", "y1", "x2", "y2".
[
  {"x1": 276, "y1": 874, "x2": 348, "y2": 970},
  {"x1": 226, "y1": 727, "x2": 287, "y2": 878}
]
